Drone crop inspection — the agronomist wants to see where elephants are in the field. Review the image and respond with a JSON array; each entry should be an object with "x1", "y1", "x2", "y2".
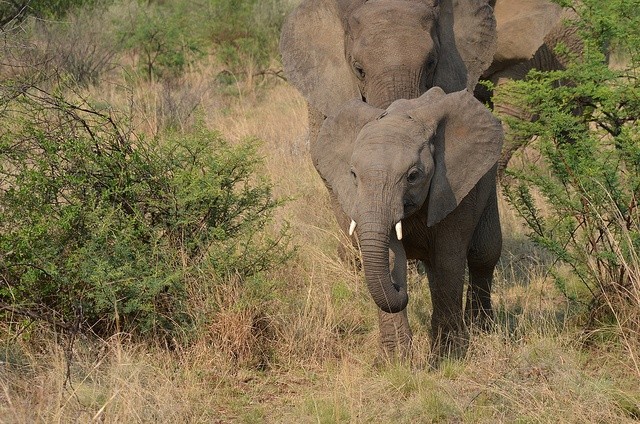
[
  {"x1": 490, "y1": 0, "x2": 610, "y2": 200},
  {"x1": 278, "y1": 0, "x2": 564, "y2": 276},
  {"x1": 310, "y1": 85, "x2": 504, "y2": 379}
]
